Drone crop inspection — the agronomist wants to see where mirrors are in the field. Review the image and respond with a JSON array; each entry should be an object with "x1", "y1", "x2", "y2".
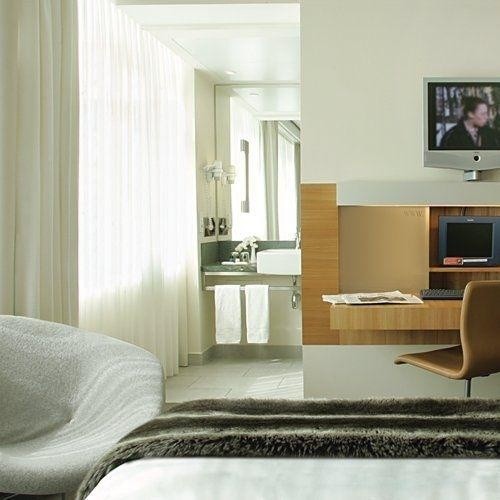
[{"x1": 212, "y1": 81, "x2": 300, "y2": 243}]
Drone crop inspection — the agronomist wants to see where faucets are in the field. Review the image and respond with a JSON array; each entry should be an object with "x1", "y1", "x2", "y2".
[{"x1": 295, "y1": 231, "x2": 301, "y2": 249}]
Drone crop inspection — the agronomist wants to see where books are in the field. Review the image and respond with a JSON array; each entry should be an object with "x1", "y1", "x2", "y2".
[
  {"x1": 341, "y1": 291, "x2": 424, "y2": 305},
  {"x1": 321, "y1": 289, "x2": 403, "y2": 305}
]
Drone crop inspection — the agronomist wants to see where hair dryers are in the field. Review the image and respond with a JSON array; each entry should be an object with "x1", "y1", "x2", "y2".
[
  {"x1": 202, "y1": 160, "x2": 224, "y2": 181},
  {"x1": 225, "y1": 165, "x2": 236, "y2": 183}
]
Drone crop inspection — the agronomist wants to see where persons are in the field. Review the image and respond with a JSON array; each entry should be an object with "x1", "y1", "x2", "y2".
[{"x1": 438, "y1": 94, "x2": 500, "y2": 147}]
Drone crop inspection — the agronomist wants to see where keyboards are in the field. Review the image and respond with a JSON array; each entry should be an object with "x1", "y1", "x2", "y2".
[{"x1": 422, "y1": 289, "x2": 464, "y2": 300}]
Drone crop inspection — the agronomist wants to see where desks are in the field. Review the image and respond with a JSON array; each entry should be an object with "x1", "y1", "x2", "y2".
[{"x1": 321, "y1": 290, "x2": 467, "y2": 336}]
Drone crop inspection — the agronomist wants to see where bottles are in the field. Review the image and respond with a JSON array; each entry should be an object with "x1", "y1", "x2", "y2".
[
  {"x1": 220, "y1": 218, "x2": 227, "y2": 234},
  {"x1": 205, "y1": 218, "x2": 215, "y2": 236}
]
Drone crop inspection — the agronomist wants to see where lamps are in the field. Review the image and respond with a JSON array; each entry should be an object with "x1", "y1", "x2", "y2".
[
  {"x1": 205, "y1": 159, "x2": 223, "y2": 185},
  {"x1": 222, "y1": 165, "x2": 237, "y2": 189}
]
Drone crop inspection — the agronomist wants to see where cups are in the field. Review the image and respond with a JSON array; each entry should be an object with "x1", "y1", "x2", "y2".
[
  {"x1": 230, "y1": 252, "x2": 239, "y2": 263},
  {"x1": 241, "y1": 252, "x2": 249, "y2": 263}
]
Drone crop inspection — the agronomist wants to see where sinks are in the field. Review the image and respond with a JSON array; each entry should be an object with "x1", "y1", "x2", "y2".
[{"x1": 256, "y1": 248, "x2": 301, "y2": 276}]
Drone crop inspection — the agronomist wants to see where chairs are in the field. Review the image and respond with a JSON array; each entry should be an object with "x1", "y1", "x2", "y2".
[
  {"x1": 0, "y1": 307, "x2": 171, "y2": 499},
  {"x1": 394, "y1": 276, "x2": 499, "y2": 400}
]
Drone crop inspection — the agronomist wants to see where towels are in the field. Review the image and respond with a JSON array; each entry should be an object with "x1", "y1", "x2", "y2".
[
  {"x1": 243, "y1": 283, "x2": 273, "y2": 345},
  {"x1": 213, "y1": 283, "x2": 243, "y2": 347}
]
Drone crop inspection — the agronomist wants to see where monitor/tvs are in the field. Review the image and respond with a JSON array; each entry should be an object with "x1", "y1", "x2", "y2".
[
  {"x1": 437, "y1": 214, "x2": 500, "y2": 266},
  {"x1": 421, "y1": 76, "x2": 500, "y2": 181}
]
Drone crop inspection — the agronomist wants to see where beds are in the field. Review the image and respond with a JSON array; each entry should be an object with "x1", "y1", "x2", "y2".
[{"x1": 75, "y1": 397, "x2": 499, "y2": 500}]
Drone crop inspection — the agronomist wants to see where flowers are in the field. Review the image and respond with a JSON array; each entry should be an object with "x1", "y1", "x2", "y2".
[{"x1": 232, "y1": 231, "x2": 259, "y2": 252}]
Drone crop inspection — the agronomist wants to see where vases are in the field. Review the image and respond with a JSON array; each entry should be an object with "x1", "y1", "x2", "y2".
[{"x1": 250, "y1": 247, "x2": 257, "y2": 264}]
